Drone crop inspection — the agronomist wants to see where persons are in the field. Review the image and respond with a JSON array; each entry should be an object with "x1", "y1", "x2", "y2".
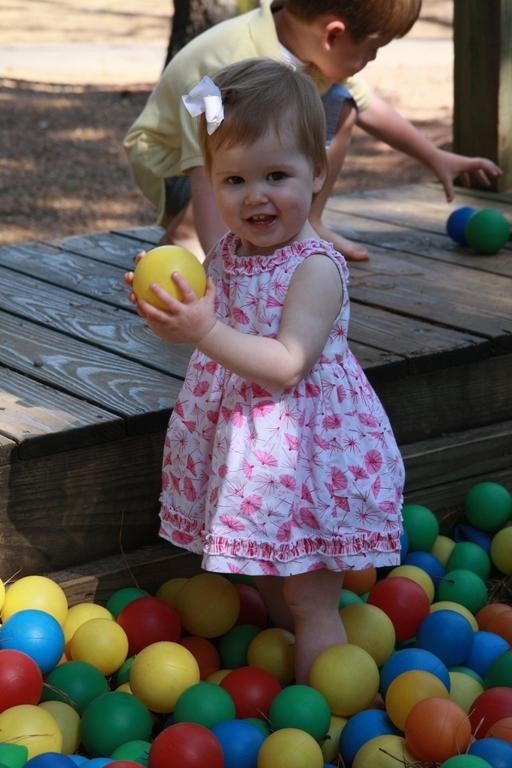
[
  {"x1": 125, "y1": 0, "x2": 505, "y2": 261},
  {"x1": 123, "y1": 58, "x2": 406, "y2": 680}
]
